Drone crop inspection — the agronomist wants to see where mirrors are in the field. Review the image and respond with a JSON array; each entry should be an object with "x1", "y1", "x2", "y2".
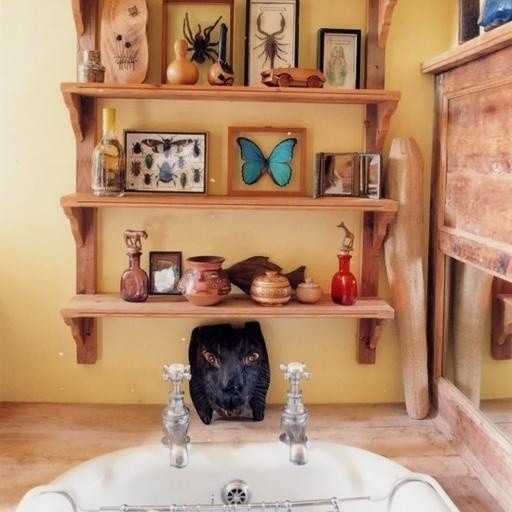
[{"x1": 442, "y1": 255, "x2": 512, "y2": 448}]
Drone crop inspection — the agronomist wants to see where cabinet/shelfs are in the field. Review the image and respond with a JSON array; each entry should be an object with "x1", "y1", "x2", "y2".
[
  {"x1": 421, "y1": 22, "x2": 512, "y2": 512},
  {"x1": 61, "y1": 1, "x2": 403, "y2": 366}
]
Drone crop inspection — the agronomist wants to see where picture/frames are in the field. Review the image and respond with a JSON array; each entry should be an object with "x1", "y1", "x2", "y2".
[
  {"x1": 160, "y1": 0, "x2": 235, "y2": 84},
  {"x1": 149, "y1": 250, "x2": 183, "y2": 295},
  {"x1": 227, "y1": 126, "x2": 308, "y2": 197},
  {"x1": 244, "y1": 0, "x2": 300, "y2": 87},
  {"x1": 121, "y1": 127, "x2": 210, "y2": 198},
  {"x1": 317, "y1": 27, "x2": 362, "y2": 90}
]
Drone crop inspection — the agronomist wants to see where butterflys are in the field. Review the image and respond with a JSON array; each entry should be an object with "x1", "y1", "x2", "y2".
[{"x1": 233, "y1": 136, "x2": 296, "y2": 186}]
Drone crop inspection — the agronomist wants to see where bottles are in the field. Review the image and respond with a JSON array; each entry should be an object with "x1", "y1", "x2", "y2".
[
  {"x1": 91, "y1": 107, "x2": 125, "y2": 198},
  {"x1": 333, "y1": 254, "x2": 357, "y2": 305},
  {"x1": 121, "y1": 249, "x2": 148, "y2": 302}
]
